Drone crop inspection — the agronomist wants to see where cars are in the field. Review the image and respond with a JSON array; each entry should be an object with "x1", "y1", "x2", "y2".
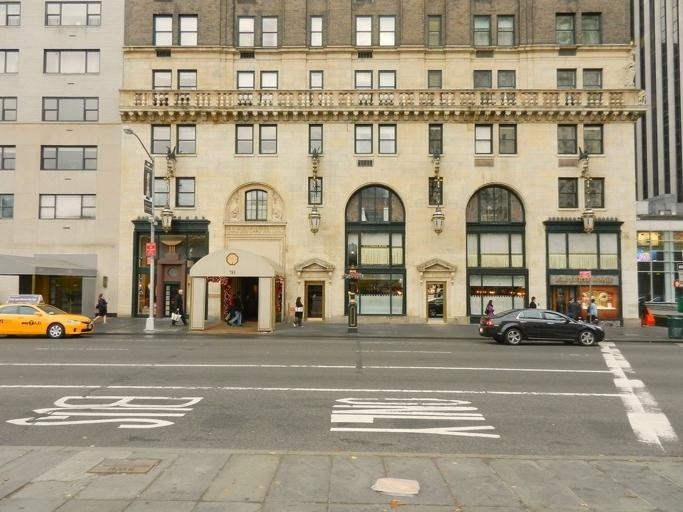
[
  {"x1": 0, "y1": 294, "x2": 95, "y2": 338},
  {"x1": 428, "y1": 298, "x2": 443, "y2": 318},
  {"x1": 478, "y1": 308, "x2": 605, "y2": 346}
]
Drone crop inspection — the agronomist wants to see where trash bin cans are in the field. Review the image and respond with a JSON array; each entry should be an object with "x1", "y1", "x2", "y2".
[{"x1": 667, "y1": 315, "x2": 683, "y2": 339}]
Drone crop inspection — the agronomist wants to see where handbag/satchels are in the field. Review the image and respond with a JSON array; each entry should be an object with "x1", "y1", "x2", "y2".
[{"x1": 171, "y1": 313, "x2": 182, "y2": 321}]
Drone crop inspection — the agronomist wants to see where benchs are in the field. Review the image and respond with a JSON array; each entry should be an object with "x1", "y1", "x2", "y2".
[{"x1": 642, "y1": 302, "x2": 683, "y2": 324}]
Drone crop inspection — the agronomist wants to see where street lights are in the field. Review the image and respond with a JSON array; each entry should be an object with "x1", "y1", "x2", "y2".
[{"x1": 122, "y1": 126, "x2": 158, "y2": 331}]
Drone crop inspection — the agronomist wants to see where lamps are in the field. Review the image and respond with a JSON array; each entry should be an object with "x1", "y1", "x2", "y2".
[
  {"x1": 432, "y1": 157, "x2": 446, "y2": 237},
  {"x1": 122, "y1": 125, "x2": 155, "y2": 164},
  {"x1": 308, "y1": 157, "x2": 322, "y2": 233},
  {"x1": 160, "y1": 145, "x2": 176, "y2": 233},
  {"x1": 578, "y1": 146, "x2": 595, "y2": 235}
]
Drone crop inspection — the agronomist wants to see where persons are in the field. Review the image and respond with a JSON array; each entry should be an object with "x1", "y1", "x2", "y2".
[
  {"x1": 224, "y1": 295, "x2": 236, "y2": 322},
  {"x1": 486, "y1": 300, "x2": 494, "y2": 317},
  {"x1": 93, "y1": 293, "x2": 108, "y2": 324},
  {"x1": 638, "y1": 299, "x2": 649, "y2": 327},
  {"x1": 227, "y1": 291, "x2": 243, "y2": 326},
  {"x1": 530, "y1": 297, "x2": 536, "y2": 308},
  {"x1": 567, "y1": 297, "x2": 581, "y2": 321},
  {"x1": 171, "y1": 288, "x2": 188, "y2": 326},
  {"x1": 293, "y1": 297, "x2": 304, "y2": 328},
  {"x1": 589, "y1": 299, "x2": 598, "y2": 325}
]
